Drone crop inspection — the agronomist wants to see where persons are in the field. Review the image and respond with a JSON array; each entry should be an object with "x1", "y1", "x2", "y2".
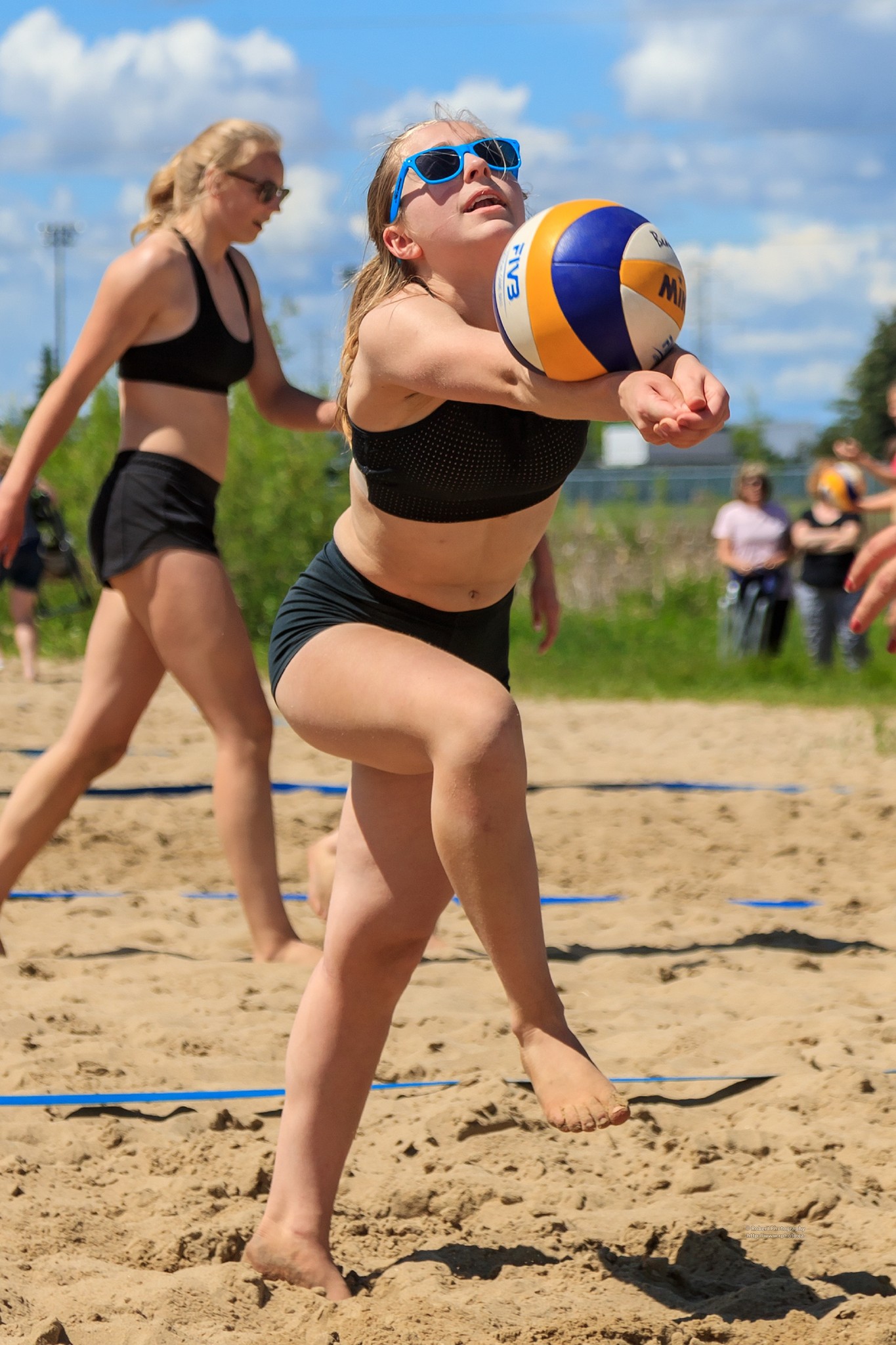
[
  {"x1": 710, "y1": 379, "x2": 896, "y2": 675},
  {"x1": 0, "y1": 449, "x2": 84, "y2": 684},
  {"x1": 0, "y1": 118, "x2": 349, "y2": 970},
  {"x1": 229, "y1": 115, "x2": 733, "y2": 1304}
]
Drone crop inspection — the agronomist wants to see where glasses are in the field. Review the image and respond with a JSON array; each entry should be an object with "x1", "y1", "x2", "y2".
[
  {"x1": 389, "y1": 130, "x2": 522, "y2": 222},
  {"x1": 226, "y1": 168, "x2": 291, "y2": 207},
  {"x1": 750, "y1": 482, "x2": 760, "y2": 487}
]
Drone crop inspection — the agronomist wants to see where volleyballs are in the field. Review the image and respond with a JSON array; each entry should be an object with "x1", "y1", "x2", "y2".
[
  {"x1": 494, "y1": 195, "x2": 685, "y2": 381},
  {"x1": 819, "y1": 462, "x2": 866, "y2": 511}
]
